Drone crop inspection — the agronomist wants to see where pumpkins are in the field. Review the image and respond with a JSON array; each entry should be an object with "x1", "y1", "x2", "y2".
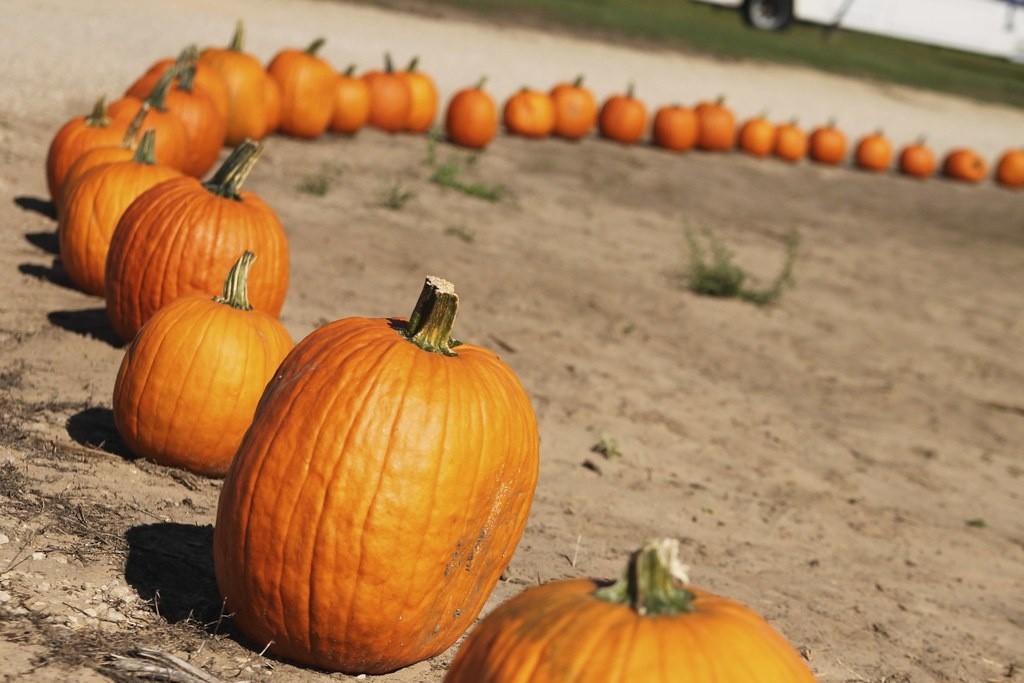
[{"x1": 47, "y1": 19, "x2": 1024, "y2": 683}]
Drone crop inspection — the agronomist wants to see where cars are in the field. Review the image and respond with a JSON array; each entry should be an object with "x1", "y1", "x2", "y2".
[{"x1": 701, "y1": 0, "x2": 1024, "y2": 72}]
